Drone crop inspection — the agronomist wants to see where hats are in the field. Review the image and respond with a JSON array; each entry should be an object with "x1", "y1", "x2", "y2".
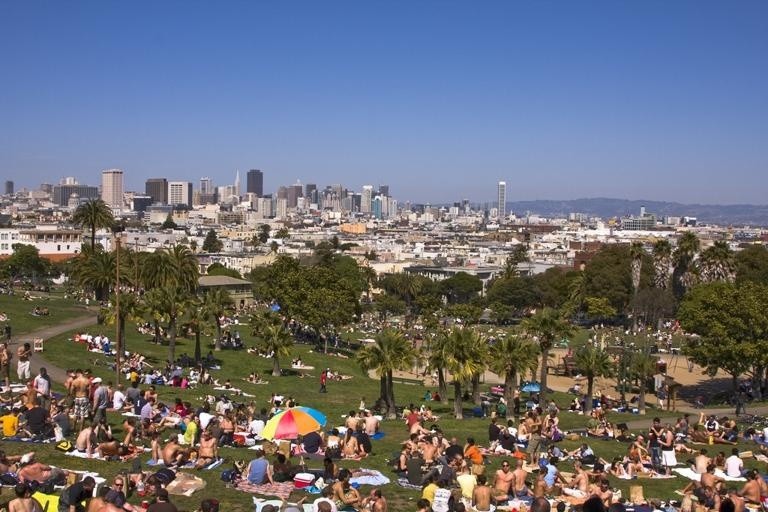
[{"x1": 261, "y1": 504, "x2": 279, "y2": 512}]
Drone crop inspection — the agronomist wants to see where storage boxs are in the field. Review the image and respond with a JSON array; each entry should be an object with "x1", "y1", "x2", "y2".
[{"x1": 293, "y1": 473, "x2": 315, "y2": 490}]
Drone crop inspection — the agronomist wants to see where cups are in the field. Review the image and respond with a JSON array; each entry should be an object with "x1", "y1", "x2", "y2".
[{"x1": 142, "y1": 500, "x2": 149, "y2": 508}]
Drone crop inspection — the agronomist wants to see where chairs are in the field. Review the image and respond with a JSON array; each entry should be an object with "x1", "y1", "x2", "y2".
[
  {"x1": 617, "y1": 423, "x2": 628, "y2": 434},
  {"x1": 629, "y1": 485, "x2": 648, "y2": 506}
]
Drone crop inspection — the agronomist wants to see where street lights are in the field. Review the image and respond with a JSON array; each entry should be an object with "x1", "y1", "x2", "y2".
[
  {"x1": 109, "y1": 222, "x2": 127, "y2": 390},
  {"x1": 133, "y1": 237, "x2": 139, "y2": 291}
]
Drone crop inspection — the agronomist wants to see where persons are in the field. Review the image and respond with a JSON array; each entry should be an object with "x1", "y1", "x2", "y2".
[
  {"x1": 61, "y1": 282, "x2": 147, "y2": 310},
  {"x1": 393, "y1": 374, "x2": 768, "y2": 511},
  {"x1": 2, "y1": 273, "x2": 50, "y2": 317},
  {"x1": 137, "y1": 298, "x2": 276, "y2": 349},
  {"x1": 280, "y1": 311, "x2": 691, "y2": 353},
  {"x1": 0, "y1": 313, "x2": 387, "y2": 511}
]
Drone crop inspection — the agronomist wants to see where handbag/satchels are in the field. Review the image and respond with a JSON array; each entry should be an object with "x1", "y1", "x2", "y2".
[
  {"x1": 221, "y1": 460, "x2": 245, "y2": 481},
  {"x1": 154, "y1": 468, "x2": 177, "y2": 485},
  {"x1": 56, "y1": 440, "x2": 74, "y2": 451},
  {"x1": 552, "y1": 432, "x2": 559, "y2": 441},
  {"x1": 0, "y1": 474, "x2": 16, "y2": 485}
]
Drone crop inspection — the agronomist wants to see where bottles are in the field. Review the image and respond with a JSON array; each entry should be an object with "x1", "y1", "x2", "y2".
[{"x1": 136, "y1": 479, "x2": 145, "y2": 498}]
